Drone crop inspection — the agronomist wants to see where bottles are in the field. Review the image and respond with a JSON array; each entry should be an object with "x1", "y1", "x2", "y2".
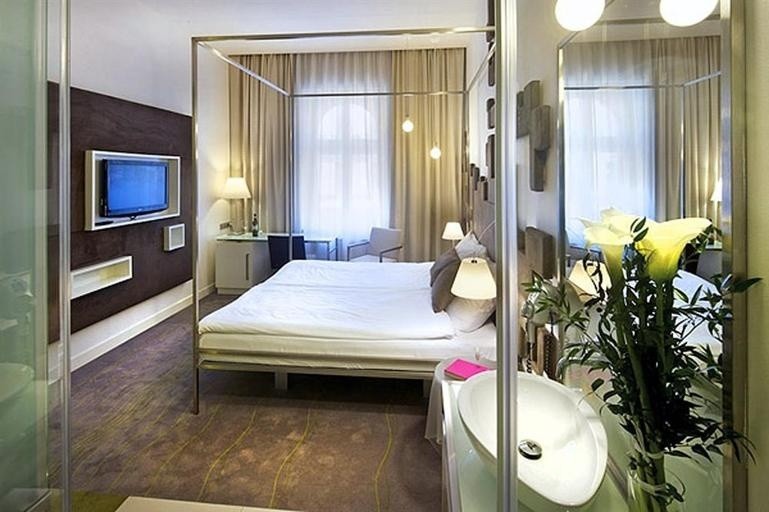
[
  {"x1": 252, "y1": 213, "x2": 259, "y2": 237},
  {"x1": 708, "y1": 219, "x2": 714, "y2": 245}
]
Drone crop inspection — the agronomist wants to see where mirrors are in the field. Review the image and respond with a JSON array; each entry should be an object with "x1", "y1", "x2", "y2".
[{"x1": 557, "y1": 0, "x2": 747, "y2": 512}]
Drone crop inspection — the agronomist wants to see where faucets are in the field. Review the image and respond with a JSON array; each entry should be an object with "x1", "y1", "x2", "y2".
[{"x1": 554, "y1": 356, "x2": 609, "y2": 382}]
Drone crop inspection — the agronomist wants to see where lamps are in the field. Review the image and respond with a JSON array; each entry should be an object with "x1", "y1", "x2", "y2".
[
  {"x1": 442, "y1": 222, "x2": 464, "y2": 247},
  {"x1": 402, "y1": 114, "x2": 414, "y2": 132},
  {"x1": 429, "y1": 142, "x2": 441, "y2": 160},
  {"x1": 223, "y1": 177, "x2": 252, "y2": 236},
  {"x1": 450, "y1": 257, "x2": 497, "y2": 363}
]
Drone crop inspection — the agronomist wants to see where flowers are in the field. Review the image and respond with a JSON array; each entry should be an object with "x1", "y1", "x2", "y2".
[{"x1": 522, "y1": 209, "x2": 762, "y2": 512}]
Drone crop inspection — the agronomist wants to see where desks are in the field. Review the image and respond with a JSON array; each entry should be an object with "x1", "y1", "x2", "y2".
[{"x1": 214, "y1": 232, "x2": 338, "y2": 295}]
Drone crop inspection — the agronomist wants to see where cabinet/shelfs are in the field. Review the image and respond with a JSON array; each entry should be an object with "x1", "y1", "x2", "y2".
[{"x1": 697, "y1": 240, "x2": 722, "y2": 276}]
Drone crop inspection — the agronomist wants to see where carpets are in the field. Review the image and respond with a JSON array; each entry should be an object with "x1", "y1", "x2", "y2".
[{"x1": 114, "y1": 495, "x2": 300, "y2": 512}]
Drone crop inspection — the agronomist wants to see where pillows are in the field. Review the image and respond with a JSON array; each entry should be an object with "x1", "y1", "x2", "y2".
[{"x1": 429, "y1": 229, "x2": 497, "y2": 332}]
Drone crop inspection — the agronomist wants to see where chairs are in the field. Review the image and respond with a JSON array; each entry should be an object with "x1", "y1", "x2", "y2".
[
  {"x1": 268, "y1": 235, "x2": 305, "y2": 269},
  {"x1": 347, "y1": 227, "x2": 402, "y2": 262}
]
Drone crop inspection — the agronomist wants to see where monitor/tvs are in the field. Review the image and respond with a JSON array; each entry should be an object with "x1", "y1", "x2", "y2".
[{"x1": 99, "y1": 158, "x2": 169, "y2": 220}]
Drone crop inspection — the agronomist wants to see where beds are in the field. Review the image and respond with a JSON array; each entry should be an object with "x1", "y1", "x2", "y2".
[{"x1": 191, "y1": 27, "x2": 497, "y2": 414}]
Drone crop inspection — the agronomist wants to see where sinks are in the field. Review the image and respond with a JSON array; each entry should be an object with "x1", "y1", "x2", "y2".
[
  {"x1": 0, "y1": 362, "x2": 34, "y2": 407},
  {"x1": 457, "y1": 368, "x2": 608, "y2": 511}
]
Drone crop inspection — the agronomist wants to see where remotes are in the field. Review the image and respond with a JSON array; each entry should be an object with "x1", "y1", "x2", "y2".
[{"x1": 95, "y1": 220, "x2": 114, "y2": 225}]
